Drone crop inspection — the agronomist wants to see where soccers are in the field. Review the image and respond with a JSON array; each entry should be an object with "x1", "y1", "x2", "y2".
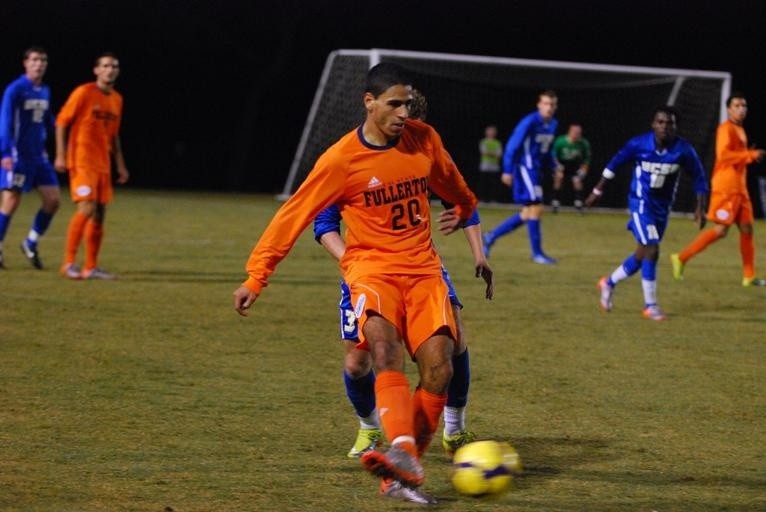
[{"x1": 451, "y1": 439, "x2": 522, "y2": 498}]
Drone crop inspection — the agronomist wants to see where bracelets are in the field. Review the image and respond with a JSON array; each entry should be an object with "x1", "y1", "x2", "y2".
[{"x1": 591, "y1": 187, "x2": 604, "y2": 197}]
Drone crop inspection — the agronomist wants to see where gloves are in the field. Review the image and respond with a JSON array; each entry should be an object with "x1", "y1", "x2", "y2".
[{"x1": 555, "y1": 163, "x2": 585, "y2": 183}]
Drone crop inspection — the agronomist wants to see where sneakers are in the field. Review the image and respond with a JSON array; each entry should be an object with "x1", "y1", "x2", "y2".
[
  {"x1": 640, "y1": 304, "x2": 673, "y2": 323},
  {"x1": 359, "y1": 446, "x2": 425, "y2": 488},
  {"x1": 529, "y1": 253, "x2": 560, "y2": 264},
  {"x1": 347, "y1": 427, "x2": 384, "y2": 460},
  {"x1": 597, "y1": 274, "x2": 615, "y2": 312},
  {"x1": 58, "y1": 262, "x2": 82, "y2": 280},
  {"x1": 19, "y1": 238, "x2": 44, "y2": 272},
  {"x1": 82, "y1": 268, "x2": 118, "y2": 281},
  {"x1": 378, "y1": 476, "x2": 440, "y2": 507},
  {"x1": 741, "y1": 277, "x2": 766, "y2": 288},
  {"x1": 551, "y1": 206, "x2": 584, "y2": 214},
  {"x1": 668, "y1": 253, "x2": 686, "y2": 284},
  {"x1": 481, "y1": 228, "x2": 496, "y2": 258},
  {"x1": 441, "y1": 426, "x2": 478, "y2": 454}
]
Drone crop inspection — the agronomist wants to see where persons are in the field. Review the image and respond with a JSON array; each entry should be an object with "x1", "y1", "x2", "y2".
[
  {"x1": 0, "y1": 46, "x2": 61, "y2": 270},
  {"x1": 53, "y1": 51, "x2": 131, "y2": 281},
  {"x1": 232, "y1": 61, "x2": 478, "y2": 504},
  {"x1": 668, "y1": 92, "x2": 766, "y2": 287},
  {"x1": 550, "y1": 120, "x2": 592, "y2": 214},
  {"x1": 313, "y1": 89, "x2": 494, "y2": 457},
  {"x1": 481, "y1": 87, "x2": 560, "y2": 265},
  {"x1": 475, "y1": 123, "x2": 508, "y2": 203},
  {"x1": 584, "y1": 105, "x2": 711, "y2": 321}
]
